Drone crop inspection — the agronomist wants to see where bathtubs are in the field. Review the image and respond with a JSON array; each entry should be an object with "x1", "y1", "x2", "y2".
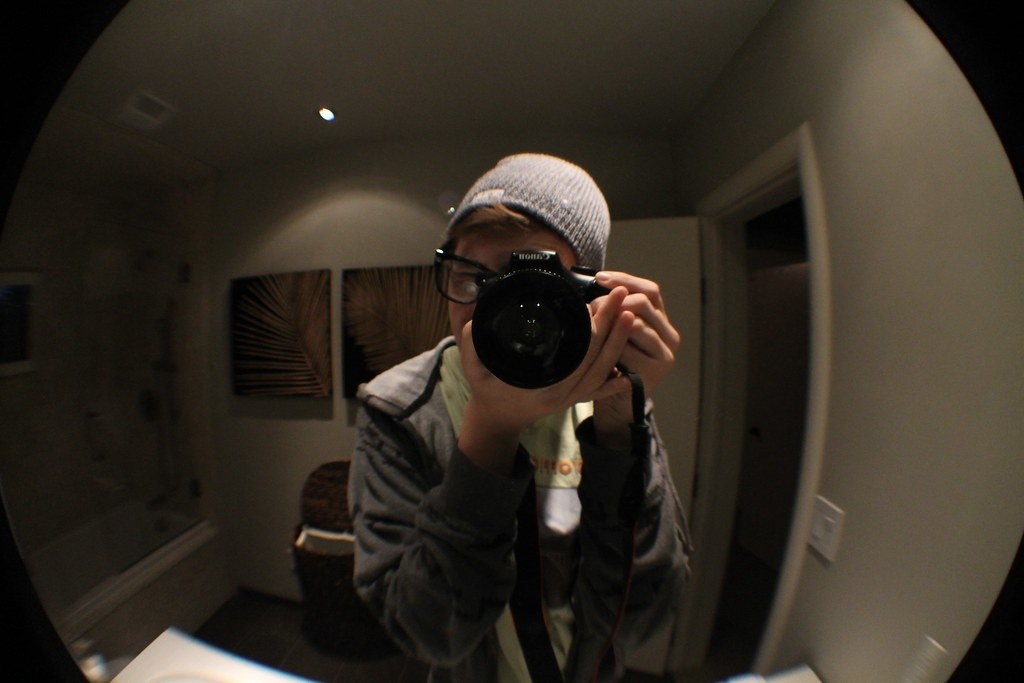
[{"x1": 21, "y1": 500, "x2": 227, "y2": 683}]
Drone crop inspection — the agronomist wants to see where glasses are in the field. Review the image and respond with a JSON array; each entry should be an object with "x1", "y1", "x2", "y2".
[{"x1": 434, "y1": 247, "x2": 500, "y2": 305}]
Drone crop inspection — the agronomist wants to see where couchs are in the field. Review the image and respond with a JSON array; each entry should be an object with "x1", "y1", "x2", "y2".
[{"x1": 293, "y1": 460, "x2": 386, "y2": 647}]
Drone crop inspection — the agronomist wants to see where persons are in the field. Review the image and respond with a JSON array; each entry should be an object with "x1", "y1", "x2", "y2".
[{"x1": 346, "y1": 151, "x2": 696, "y2": 683}]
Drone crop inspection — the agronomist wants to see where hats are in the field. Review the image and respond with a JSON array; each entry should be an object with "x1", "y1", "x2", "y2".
[{"x1": 442, "y1": 153, "x2": 611, "y2": 272}]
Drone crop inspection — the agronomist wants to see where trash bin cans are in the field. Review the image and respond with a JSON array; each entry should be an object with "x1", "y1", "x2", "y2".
[{"x1": 290, "y1": 523, "x2": 403, "y2": 662}]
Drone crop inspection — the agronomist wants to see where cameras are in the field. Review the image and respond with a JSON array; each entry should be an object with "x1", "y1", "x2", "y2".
[{"x1": 472, "y1": 249, "x2": 612, "y2": 389}]
